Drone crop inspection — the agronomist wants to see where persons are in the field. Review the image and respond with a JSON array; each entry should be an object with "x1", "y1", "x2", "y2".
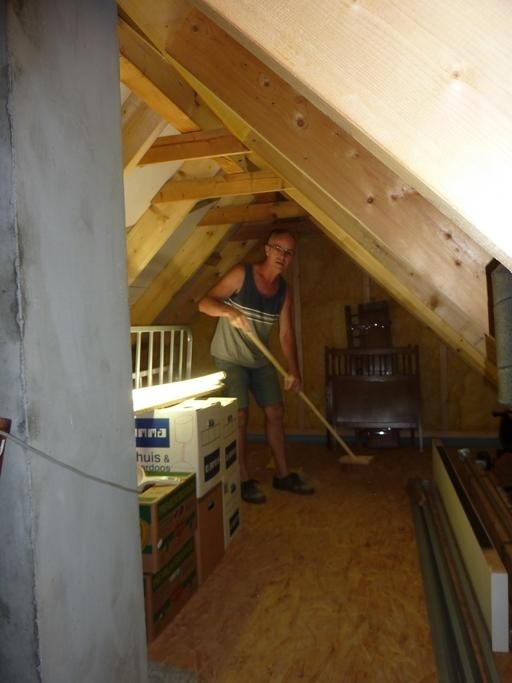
[{"x1": 199, "y1": 227, "x2": 315, "y2": 504}]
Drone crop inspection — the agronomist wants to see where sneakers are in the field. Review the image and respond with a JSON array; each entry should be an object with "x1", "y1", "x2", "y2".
[
  {"x1": 273, "y1": 471, "x2": 315, "y2": 495},
  {"x1": 241, "y1": 478, "x2": 266, "y2": 503}
]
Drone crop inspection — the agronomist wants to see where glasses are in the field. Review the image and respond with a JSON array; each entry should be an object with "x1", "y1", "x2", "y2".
[{"x1": 267, "y1": 244, "x2": 296, "y2": 257}]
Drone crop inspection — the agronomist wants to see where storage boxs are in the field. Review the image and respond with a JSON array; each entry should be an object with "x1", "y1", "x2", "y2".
[{"x1": 123, "y1": 395, "x2": 245, "y2": 644}]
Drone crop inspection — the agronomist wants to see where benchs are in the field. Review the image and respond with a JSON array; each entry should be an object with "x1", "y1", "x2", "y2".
[{"x1": 321, "y1": 344, "x2": 427, "y2": 455}]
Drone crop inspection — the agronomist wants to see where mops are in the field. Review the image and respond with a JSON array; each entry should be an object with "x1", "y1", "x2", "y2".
[{"x1": 244, "y1": 326, "x2": 375, "y2": 478}]
